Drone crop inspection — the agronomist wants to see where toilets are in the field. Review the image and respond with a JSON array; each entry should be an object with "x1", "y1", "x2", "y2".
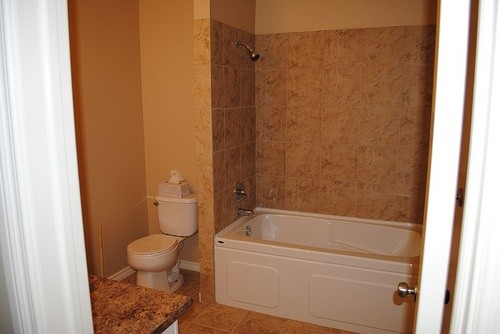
[{"x1": 127, "y1": 195, "x2": 198, "y2": 292}]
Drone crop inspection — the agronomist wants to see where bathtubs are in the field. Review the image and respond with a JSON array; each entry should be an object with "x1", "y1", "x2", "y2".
[{"x1": 214, "y1": 207, "x2": 422, "y2": 334}]
